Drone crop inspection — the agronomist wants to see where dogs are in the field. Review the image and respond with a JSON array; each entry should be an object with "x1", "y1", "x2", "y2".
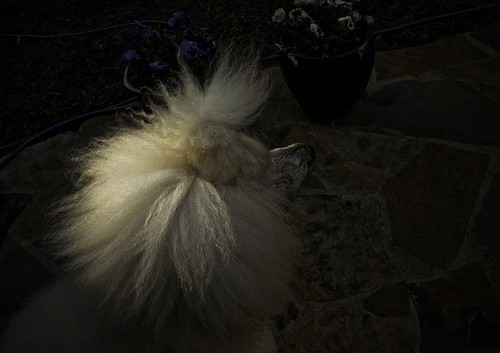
[{"x1": 1, "y1": 37, "x2": 316, "y2": 353}]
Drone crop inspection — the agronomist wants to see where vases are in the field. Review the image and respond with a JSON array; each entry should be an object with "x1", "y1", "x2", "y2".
[{"x1": 279, "y1": 20, "x2": 375, "y2": 124}]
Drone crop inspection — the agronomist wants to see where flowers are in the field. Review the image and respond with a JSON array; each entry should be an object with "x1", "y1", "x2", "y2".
[
  {"x1": 121, "y1": 11, "x2": 222, "y2": 89},
  {"x1": 269, "y1": 0, "x2": 375, "y2": 67}
]
